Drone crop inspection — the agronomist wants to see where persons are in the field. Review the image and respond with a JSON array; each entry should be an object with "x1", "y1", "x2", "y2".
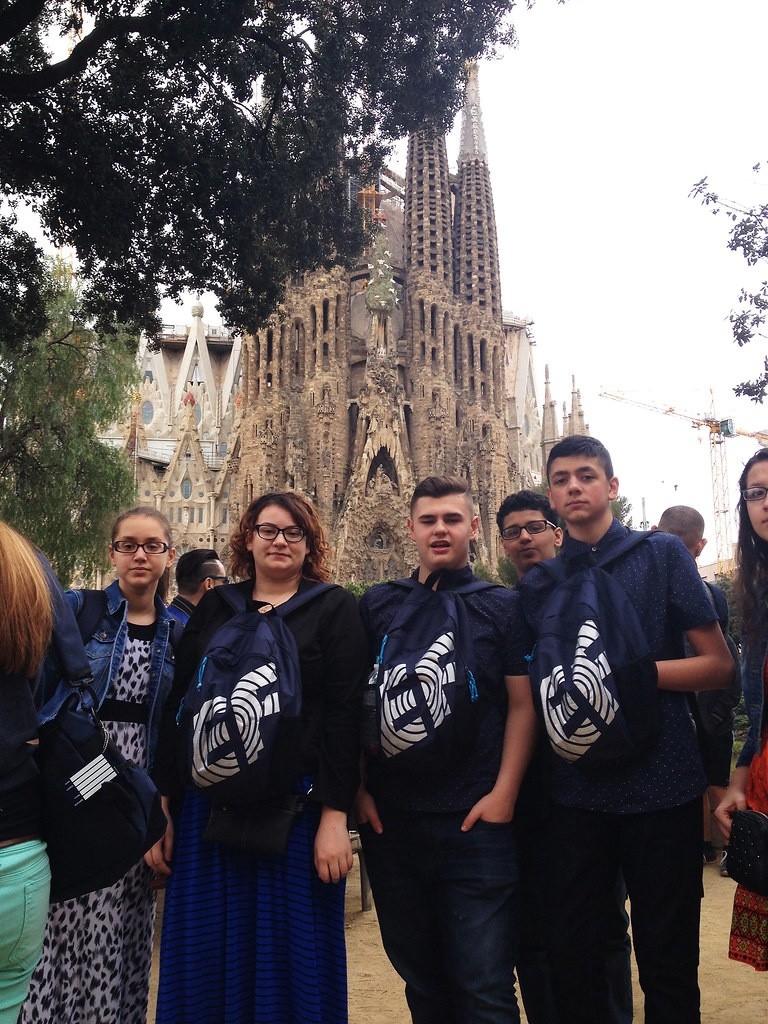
[
  {"x1": 358, "y1": 475, "x2": 542, "y2": 1024},
  {"x1": 714, "y1": 448, "x2": 768, "y2": 972},
  {"x1": 167, "y1": 549, "x2": 230, "y2": 626},
  {"x1": 17, "y1": 506, "x2": 187, "y2": 1024},
  {"x1": 651, "y1": 505, "x2": 734, "y2": 876},
  {"x1": 497, "y1": 489, "x2": 563, "y2": 579},
  {"x1": 0, "y1": 521, "x2": 93, "y2": 1024},
  {"x1": 144, "y1": 491, "x2": 368, "y2": 1024},
  {"x1": 513, "y1": 434, "x2": 736, "y2": 1024}
]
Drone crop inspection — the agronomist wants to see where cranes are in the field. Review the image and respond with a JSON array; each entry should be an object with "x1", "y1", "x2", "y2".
[{"x1": 599, "y1": 384, "x2": 768, "y2": 581}]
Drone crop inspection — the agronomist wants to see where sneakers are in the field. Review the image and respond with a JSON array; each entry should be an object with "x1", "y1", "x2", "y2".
[
  {"x1": 702, "y1": 843, "x2": 718, "y2": 864},
  {"x1": 719, "y1": 850, "x2": 728, "y2": 877}
]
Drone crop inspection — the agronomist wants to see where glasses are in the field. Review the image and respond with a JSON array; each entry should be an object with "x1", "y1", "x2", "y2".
[
  {"x1": 742, "y1": 487, "x2": 768, "y2": 501},
  {"x1": 113, "y1": 540, "x2": 170, "y2": 554},
  {"x1": 250, "y1": 524, "x2": 308, "y2": 542},
  {"x1": 500, "y1": 520, "x2": 557, "y2": 541},
  {"x1": 200, "y1": 576, "x2": 229, "y2": 585}
]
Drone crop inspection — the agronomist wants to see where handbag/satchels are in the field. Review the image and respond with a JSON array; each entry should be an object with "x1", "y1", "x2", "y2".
[
  {"x1": 727, "y1": 808, "x2": 768, "y2": 897},
  {"x1": 38, "y1": 690, "x2": 167, "y2": 902}
]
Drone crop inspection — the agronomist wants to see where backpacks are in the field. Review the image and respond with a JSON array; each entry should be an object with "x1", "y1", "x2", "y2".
[
  {"x1": 184, "y1": 583, "x2": 341, "y2": 804},
  {"x1": 360, "y1": 568, "x2": 509, "y2": 770},
  {"x1": 689, "y1": 580, "x2": 741, "y2": 735},
  {"x1": 528, "y1": 531, "x2": 660, "y2": 769}
]
